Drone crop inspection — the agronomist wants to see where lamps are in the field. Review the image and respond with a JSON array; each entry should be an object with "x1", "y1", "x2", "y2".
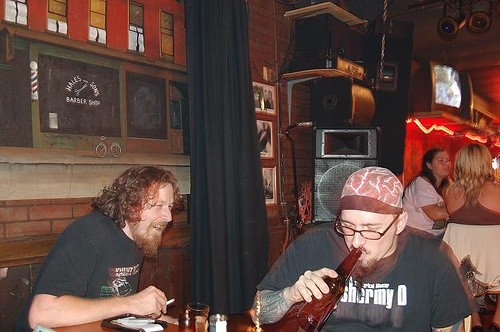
[{"x1": 437, "y1": 0, "x2": 493, "y2": 40}]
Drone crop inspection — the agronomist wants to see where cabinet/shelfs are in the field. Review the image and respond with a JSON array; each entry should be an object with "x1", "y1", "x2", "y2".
[{"x1": 282, "y1": 2, "x2": 364, "y2": 82}]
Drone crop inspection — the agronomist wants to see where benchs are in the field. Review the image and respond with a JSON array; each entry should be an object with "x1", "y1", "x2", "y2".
[{"x1": 0, "y1": 225, "x2": 297, "y2": 332}]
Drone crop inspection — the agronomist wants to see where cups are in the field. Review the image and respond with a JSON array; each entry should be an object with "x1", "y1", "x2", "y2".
[
  {"x1": 186, "y1": 303, "x2": 210, "y2": 332},
  {"x1": 208, "y1": 313, "x2": 227, "y2": 332}
]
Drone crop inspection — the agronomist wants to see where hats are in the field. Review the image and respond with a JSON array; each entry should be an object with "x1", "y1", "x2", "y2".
[{"x1": 340, "y1": 166, "x2": 403, "y2": 215}]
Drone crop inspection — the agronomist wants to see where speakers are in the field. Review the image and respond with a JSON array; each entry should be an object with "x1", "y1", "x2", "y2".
[{"x1": 311, "y1": 34, "x2": 412, "y2": 225}]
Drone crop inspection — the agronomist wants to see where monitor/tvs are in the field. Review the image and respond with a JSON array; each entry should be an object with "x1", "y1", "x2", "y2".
[{"x1": 429, "y1": 60, "x2": 469, "y2": 118}]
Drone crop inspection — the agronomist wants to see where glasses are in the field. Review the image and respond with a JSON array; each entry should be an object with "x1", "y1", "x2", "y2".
[{"x1": 333, "y1": 209, "x2": 401, "y2": 242}]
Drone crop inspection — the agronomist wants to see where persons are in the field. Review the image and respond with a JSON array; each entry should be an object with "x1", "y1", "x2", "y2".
[
  {"x1": 402, "y1": 143, "x2": 500, "y2": 332},
  {"x1": 258, "y1": 122, "x2": 270, "y2": 153},
  {"x1": 254, "y1": 89, "x2": 274, "y2": 112},
  {"x1": 250, "y1": 166, "x2": 472, "y2": 332},
  {"x1": 28, "y1": 164, "x2": 182, "y2": 329}
]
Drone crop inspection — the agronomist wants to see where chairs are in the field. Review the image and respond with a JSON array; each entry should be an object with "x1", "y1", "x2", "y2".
[{"x1": 442, "y1": 222, "x2": 500, "y2": 295}]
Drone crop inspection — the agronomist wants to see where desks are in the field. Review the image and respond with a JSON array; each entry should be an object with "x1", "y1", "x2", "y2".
[{"x1": 42, "y1": 316, "x2": 180, "y2": 332}]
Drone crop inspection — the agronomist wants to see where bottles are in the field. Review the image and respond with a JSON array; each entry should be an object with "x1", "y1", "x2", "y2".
[
  {"x1": 296, "y1": 248, "x2": 363, "y2": 332},
  {"x1": 179, "y1": 310, "x2": 197, "y2": 332}
]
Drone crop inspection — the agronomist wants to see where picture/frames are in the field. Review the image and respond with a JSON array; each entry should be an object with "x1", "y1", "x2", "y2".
[
  {"x1": 376, "y1": 62, "x2": 399, "y2": 91},
  {"x1": 252, "y1": 79, "x2": 277, "y2": 206}
]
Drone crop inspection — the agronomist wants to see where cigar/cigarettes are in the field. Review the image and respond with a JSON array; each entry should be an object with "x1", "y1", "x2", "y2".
[{"x1": 160, "y1": 298, "x2": 176, "y2": 310}]
